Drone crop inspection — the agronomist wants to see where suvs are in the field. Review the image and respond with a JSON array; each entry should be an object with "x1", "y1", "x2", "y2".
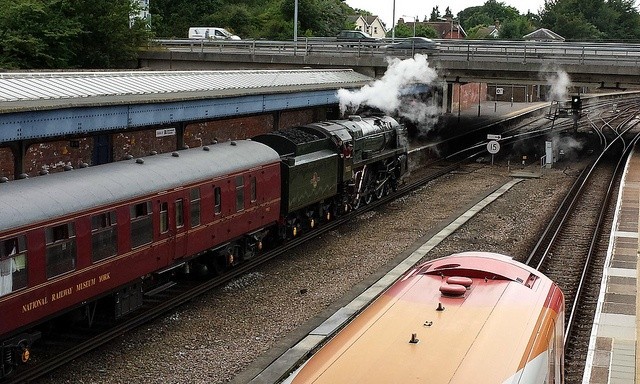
[{"x1": 336, "y1": 31, "x2": 379, "y2": 41}]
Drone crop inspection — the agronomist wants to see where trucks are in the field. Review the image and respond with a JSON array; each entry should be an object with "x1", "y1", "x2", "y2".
[{"x1": 188, "y1": 27, "x2": 241, "y2": 41}]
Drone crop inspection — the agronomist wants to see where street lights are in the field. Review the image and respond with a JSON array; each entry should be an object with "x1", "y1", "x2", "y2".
[
  {"x1": 440, "y1": 18, "x2": 460, "y2": 38},
  {"x1": 402, "y1": 15, "x2": 415, "y2": 37},
  {"x1": 436, "y1": 17, "x2": 453, "y2": 38}
]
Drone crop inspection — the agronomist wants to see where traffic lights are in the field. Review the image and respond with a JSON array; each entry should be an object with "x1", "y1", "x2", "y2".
[{"x1": 572, "y1": 95, "x2": 579, "y2": 109}]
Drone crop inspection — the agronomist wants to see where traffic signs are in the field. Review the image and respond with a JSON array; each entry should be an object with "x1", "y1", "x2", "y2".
[
  {"x1": 487, "y1": 134, "x2": 501, "y2": 140},
  {"x1": 487, "y1": 141, "x2": 500, "y2": 154},
  {"x1": 496, "y1": 88, "x2": 503, "y2": 94}
]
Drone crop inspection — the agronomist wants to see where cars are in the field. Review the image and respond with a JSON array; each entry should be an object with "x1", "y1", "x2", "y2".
[{"x1": 386, "y1": 37, "x2": 435, "y2": 49}]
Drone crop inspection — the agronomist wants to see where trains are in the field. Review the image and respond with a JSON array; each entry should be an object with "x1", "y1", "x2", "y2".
[
  {"x1": 280, "y1": 251, "x2": 566, "y2": 384},
  {"x1": 0, "y1": 113, "x2": 409, "y2": 383}
]
